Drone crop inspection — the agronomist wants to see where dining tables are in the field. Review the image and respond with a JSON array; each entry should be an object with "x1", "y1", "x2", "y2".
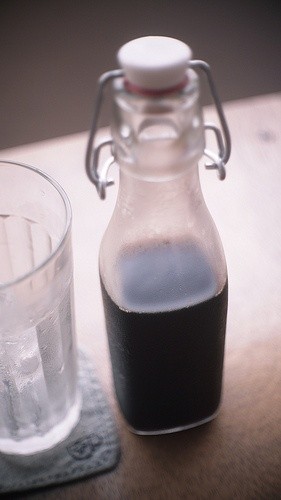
[{"x1": 0, "y1": 93, "x2": 281, "y2": 500}]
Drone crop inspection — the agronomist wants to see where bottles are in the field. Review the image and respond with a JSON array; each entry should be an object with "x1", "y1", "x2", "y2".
[{"x1": 82, "y1": 36, "x2": 229, "y2": 440}]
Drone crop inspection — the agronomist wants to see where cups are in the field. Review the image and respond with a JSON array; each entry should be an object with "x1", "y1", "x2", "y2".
[{"x1": 0, "y1": 160, "x2": 81, "y2": 461}]
